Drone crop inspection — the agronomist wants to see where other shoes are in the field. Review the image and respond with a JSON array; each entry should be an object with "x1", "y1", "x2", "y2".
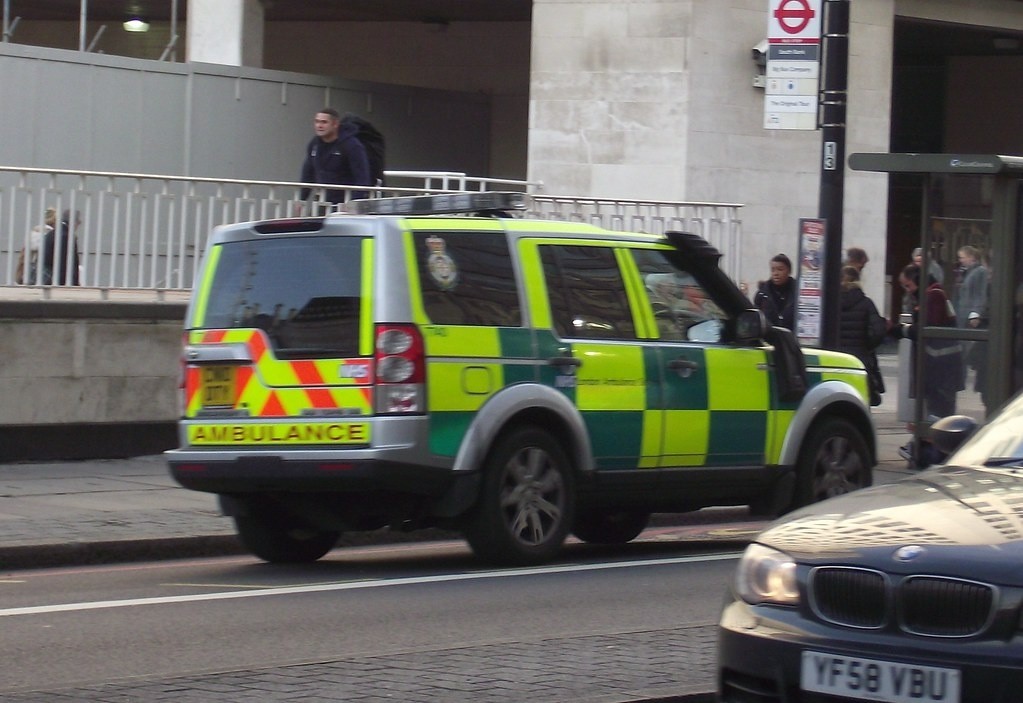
[{"x1": 898, "y1": 446, "x2": 913, "y2": 460}]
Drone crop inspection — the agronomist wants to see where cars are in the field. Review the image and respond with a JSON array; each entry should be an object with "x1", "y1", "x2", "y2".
[{"x1": 716, "y1": 388, "x2": 1023, "y2": 703}]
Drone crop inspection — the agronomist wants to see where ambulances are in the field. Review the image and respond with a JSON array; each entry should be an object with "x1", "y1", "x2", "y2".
[{"x1": 160, "y1": 189, "x2": 875, "y2": 573}]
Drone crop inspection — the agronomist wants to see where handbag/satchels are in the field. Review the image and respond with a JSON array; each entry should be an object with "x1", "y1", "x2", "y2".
[{"x1": 17, "y1": 247, "x2": 23, "y2": 284}]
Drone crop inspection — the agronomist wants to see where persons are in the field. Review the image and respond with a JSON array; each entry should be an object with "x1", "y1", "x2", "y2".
[
  {"x1": 753, "y1": 253, "x2": 796, "y2": 334},
  {"x1": 291, "y1": 108, "x2": 372, "y2": 215},
  {"x1": 838, "y1": 244, "x2": 994, "y2": 459},
  {"x1": 15, "y1": 208, "x2": 55, "y2": 285},
  {"x1": 40, "y1": 208, "x2": 81, "y2": 286}
]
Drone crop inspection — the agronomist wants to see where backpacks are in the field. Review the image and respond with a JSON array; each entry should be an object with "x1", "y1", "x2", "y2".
[{"x1": 312, "y1": 110, "x2": 385, "y2": 185}]
